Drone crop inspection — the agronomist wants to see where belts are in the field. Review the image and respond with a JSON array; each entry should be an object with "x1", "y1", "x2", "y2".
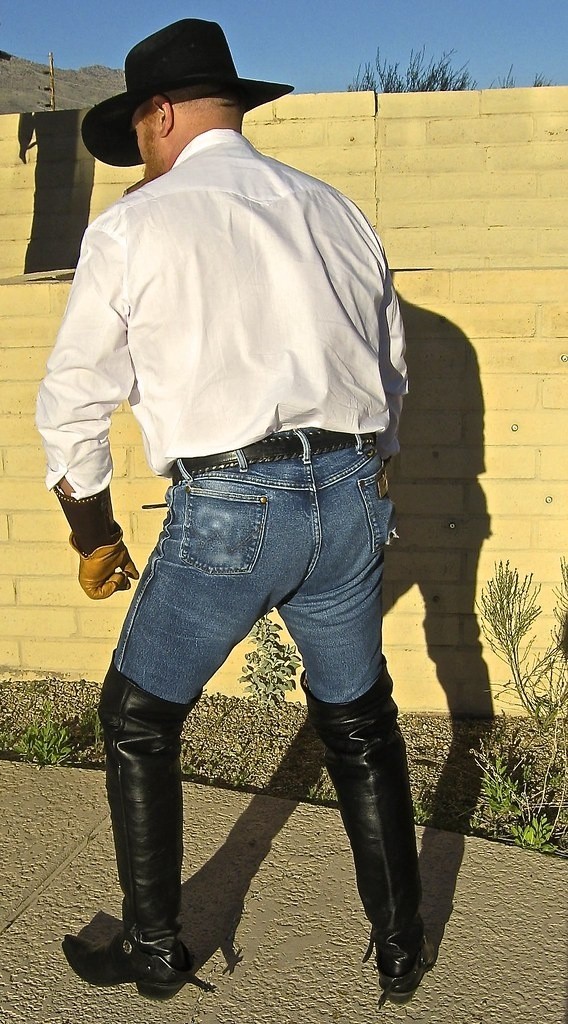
[{"x1": 171, "y1": 427, "x2": 377, "y2": 485}]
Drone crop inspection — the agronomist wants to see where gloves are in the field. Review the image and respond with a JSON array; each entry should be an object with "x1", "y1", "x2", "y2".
[{"x1": 68, "y1": 518, "x2": 139, "y2": 599}]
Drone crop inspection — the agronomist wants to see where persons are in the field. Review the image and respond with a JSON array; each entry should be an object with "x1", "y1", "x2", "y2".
[{"x1": 38, "y1": 20, "x2": 439, "y2": 1009}]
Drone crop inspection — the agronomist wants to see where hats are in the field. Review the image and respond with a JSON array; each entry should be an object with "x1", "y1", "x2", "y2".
[{"x1": 81, "y1": 18, "x2": 294, "y2": 168}]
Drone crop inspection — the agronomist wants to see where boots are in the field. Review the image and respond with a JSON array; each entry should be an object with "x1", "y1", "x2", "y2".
[
  {"x1": 299, "y1": 652, "x2": 433, "y2": 1003},
  {"x1": 62, "y1": 648, "x2": 203, "y2": 1001}
]
